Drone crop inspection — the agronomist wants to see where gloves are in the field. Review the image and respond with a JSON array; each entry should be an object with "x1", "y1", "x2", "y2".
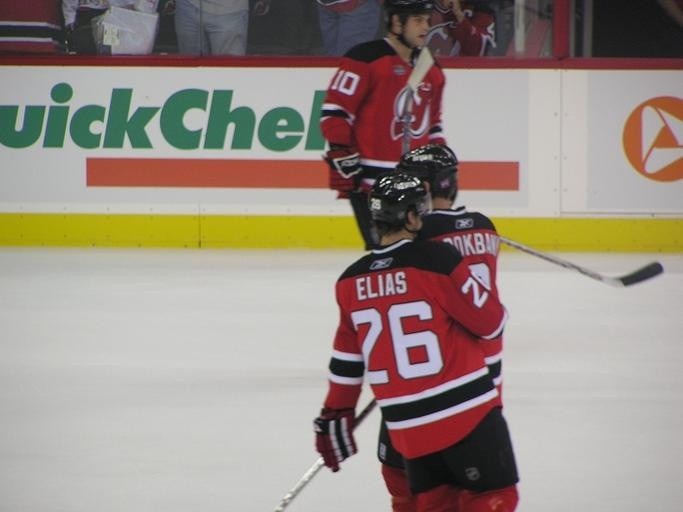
[
  {"x1": 312, "y1": 407, "x2": 359, "y2": 472},
  {"x1": 321, "y1": 148, "x2": 364, "y2": 195}
]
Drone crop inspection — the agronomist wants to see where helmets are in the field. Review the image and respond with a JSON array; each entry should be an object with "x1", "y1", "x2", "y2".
[
  {"x1": 366, "y1": 170, "x2": 426, "y2": 225},
  {"x1": 383, "y1": 1, "x2": 435, "y2": 17},
  {"x1": 396, "y1": 142, "x2": 460, "y2": 184}
]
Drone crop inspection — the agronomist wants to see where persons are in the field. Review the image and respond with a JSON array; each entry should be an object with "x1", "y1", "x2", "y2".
[
  {"x1": 63, "y1": 1, "x2": 497, "y2": 56},
  {"x1": 315, "y1": 144, "x2": 519, "y2": 512},
  {"x1": 320, "y1": 1, "x2": 446, "y2": 248}
]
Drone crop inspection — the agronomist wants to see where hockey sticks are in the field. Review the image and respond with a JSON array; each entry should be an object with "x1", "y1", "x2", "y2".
[{"x1": 500, "y1": 238, "x2": 662, "y2": 286}]
